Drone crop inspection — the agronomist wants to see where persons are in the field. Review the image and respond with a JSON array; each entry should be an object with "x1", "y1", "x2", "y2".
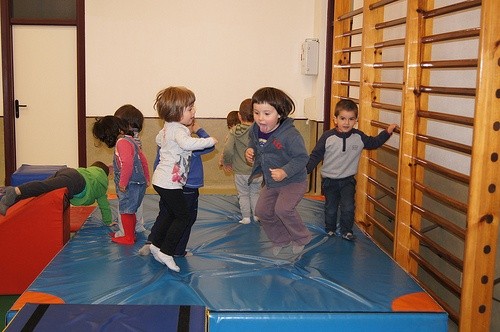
[
  {"x1": 0, "y1": 161, "x2": 119, "y2": 228},
  {"x1": 92, "y1": 105, "x2": 149, "y2": 245},
  {"x1": 218, "y1": 110, "x2": 241, "y2": 167},
  {"x1": 306, "y1": 98, "x2": 397, "y2": 240},
  {"x1": 147, "y1": 87, "x2": 217, "y2": 273},
  {"x1": 246, "y1": 87, "x2": 312, "y2": 258},
  {"x1": 138, "y1": 118, "x2": 215, "y2": 258},
  {"x1": 222, "y1": 99, "x2": 264, "y2": 225}
]
